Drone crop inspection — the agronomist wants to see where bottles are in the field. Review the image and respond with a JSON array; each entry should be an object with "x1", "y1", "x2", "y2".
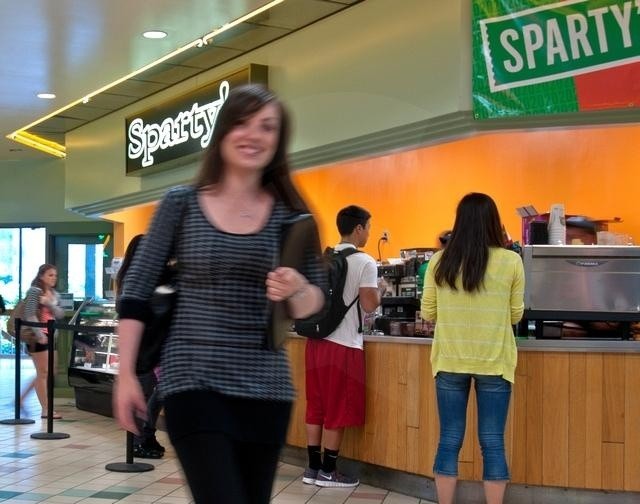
[{"x1": 390, "y1": 322, "x2": 400, "y2": 335}]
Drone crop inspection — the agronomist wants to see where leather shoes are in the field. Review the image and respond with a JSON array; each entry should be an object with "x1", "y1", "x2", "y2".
[{"x1": 133, "y1": 436, "x2": 166, "y2": 458}]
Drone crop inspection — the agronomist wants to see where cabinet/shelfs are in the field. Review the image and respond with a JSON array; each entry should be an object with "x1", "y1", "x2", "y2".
[{"x1": 68, "y1": 310, "x2": 121, "y2": 418}]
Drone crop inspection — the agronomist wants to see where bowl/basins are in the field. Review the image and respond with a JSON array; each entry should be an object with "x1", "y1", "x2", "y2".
[{"x1": 400, "y1": 322, "x2": 415, "y2": 336}]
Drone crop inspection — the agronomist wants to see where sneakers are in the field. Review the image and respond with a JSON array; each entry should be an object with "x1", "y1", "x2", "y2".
[{"x1": 302, "y1": 466, "x2": 359, "y2": 488}]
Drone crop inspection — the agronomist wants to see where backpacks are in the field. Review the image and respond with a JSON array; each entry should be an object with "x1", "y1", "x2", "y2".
[{"x1": 294, "y1": 246, "x2": 363, "y2": 339}]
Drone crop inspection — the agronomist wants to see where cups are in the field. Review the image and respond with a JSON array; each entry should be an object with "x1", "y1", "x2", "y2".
[{"x1": 529, "y1": 222, "x2": 548, "y2": 245}]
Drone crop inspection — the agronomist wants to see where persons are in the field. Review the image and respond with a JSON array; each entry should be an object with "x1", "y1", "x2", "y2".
[
  {"x1": 113, "y1": 233, "x2": 166, "y2": 459},
  {"x1": 302, "y1": 204, "x2": 387, "y2": 488},
  {"x1": 113, "y1": 83, "x2": 334, "y2": 503},
  {"x1": 414, "y1": 192, "x2": 526, "y2": 504},
  {"x1": 20, "y1": 263, "x2": 63, "y2": 420}
]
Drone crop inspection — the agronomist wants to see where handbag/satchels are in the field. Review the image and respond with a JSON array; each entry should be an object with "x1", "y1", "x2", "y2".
[
  {"x1": 7, "y1": 301, "x2": 47, "y2": 341},
  {"x1": 136, "y1": 259, "x2": 178, "y2": 375}
]
